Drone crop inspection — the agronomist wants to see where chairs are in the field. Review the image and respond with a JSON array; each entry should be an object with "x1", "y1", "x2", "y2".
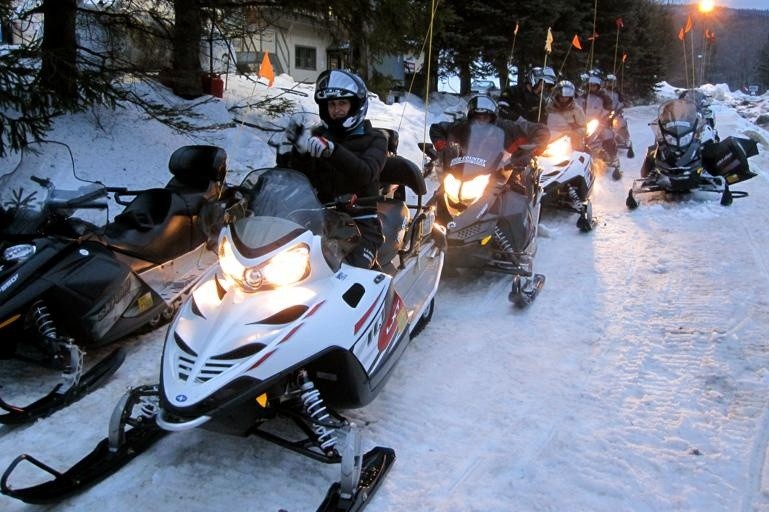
[{"x1": 166, "y1": 143, "x2": 227, "y2": 214}]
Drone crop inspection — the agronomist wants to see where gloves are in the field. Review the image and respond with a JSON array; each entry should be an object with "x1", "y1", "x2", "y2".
[{"x1": 282, "y1": 121, "x2": 334, "y2": 159}]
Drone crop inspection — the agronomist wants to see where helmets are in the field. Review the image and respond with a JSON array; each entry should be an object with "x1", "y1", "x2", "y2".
[
  {"x1": 314, "y1": 69, "x2": 370, "y2": 133},
  {"x1": 588, "y1": 77, "x2": 601, "y2": 92},
  {"x1": 604, "y1": 74, "x2": 616, "y2": 88},
  {"x1": 528, "y1": 66, "x2": 555, "y2": 88},
  {"x1": 466, "y1": 95, "x2": 499, "y2": 125},
  {"x1": 555, "y1": 81, "x2": 575, "y2": 111}
]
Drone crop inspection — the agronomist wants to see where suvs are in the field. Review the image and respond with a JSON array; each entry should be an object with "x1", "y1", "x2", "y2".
[{"x1": 234, "y1": 51, "x2": 285, "y2": 76}]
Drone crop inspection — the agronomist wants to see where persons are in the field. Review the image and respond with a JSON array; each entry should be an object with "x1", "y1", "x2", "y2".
[
  {"x1": 579, "y1": 76, "x2": 612, "y2": 126},
  {"x1": 604, "y1": 74, "x2": 624, "y2": 116},
  {"x1": 499, "y1": 67, "x2": 556, "y2": 124},
  {"x1": 266, "y1": 68, "x2": 389, "y2": 269},
  {"x1": 542, "y1": 79, "x2": 588, "y2": 151},
  {"x1": 429, "y1": 94, "x2": 551, "y2": 155}
]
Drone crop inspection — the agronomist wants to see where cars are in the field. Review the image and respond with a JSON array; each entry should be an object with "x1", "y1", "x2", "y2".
[{"x1": 470, "y1": 80, "x2": 497, "y2": 94}]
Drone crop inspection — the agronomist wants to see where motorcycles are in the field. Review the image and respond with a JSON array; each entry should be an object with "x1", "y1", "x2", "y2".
[{"x1": 628, "y1": 90, "x2": 759, "y2": 209}]
